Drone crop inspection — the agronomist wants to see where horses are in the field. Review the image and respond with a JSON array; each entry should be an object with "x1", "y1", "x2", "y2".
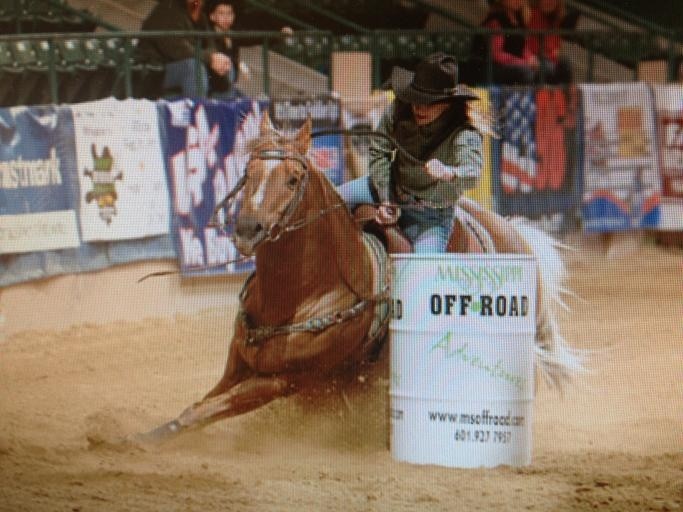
[{"x1": 143, "y1": 109, "x2": 616, "y2": 445}]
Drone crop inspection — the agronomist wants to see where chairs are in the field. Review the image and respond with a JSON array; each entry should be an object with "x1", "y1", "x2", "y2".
[{"x1": 0, "y1": 0, "x2": 168, "y2": 105}]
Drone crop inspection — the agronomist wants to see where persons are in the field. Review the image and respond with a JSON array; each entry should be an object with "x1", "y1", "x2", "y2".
[
  {"x1": 469, "y1": 0, "x2": 539, "y2": 86},
  {"x1": 200, "y1": 1, "x2": 292, "y2": 101},
  {"x1": 131, "y1": 1, "x2": 236, "y2": 101},
  {"x1": 332, "y1": 51, "x2": 483, "y2": 253},
  {"x1": 521, "y1": 0, "x2": 573, "y2": 85}
]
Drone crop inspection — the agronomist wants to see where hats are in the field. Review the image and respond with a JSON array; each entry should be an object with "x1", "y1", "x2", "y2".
[{"x1": 392, "y1": 52, "x2": 480, "y2": 103}]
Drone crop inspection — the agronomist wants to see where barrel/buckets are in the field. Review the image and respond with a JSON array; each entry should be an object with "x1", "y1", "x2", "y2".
[{"x1": 390, "y1": 253, "x2": 539, "y2": 469}]
